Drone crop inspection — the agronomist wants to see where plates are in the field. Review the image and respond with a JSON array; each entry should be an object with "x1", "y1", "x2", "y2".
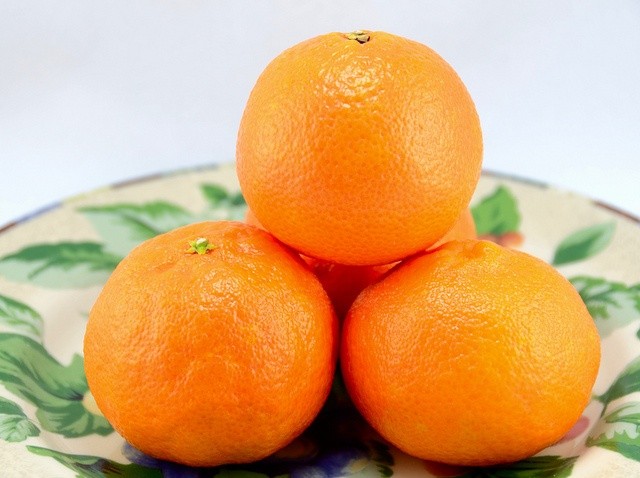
[{"x1": 0, "y1": 161, "x2": 640, "y2": 478}]
